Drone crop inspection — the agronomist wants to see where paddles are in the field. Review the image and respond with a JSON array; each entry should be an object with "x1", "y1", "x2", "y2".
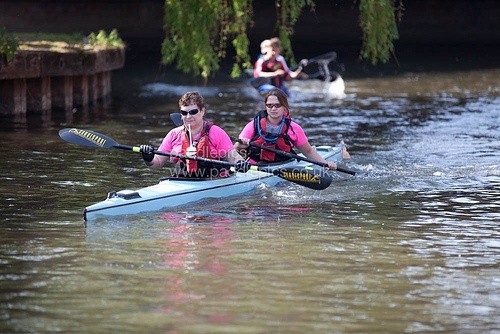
[
  {"x1": 169, "y1": 112, "x2": 357, "y2": 178},
  {"x1": 247, "y1": 51, "x2": 338, "y2": 90},
  {"x1": 57, "y1": 126, "x2": 334, "y2": 191}
]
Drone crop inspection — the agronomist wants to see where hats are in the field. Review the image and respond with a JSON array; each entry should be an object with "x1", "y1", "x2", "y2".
[{"x1": 260, "y1": 40, "x2": 271, "y2": 55}]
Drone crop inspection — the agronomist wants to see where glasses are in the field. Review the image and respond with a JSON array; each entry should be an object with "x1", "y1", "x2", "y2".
[
  {"x1": 181, "y1": 109, "x2": 198, "y2": 115},
  {"x1": 265, "y1": 102, "x2": 282, "y2": 108}
]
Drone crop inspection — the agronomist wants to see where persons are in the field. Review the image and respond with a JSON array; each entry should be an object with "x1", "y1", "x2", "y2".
[
  {"x1": 140, "y1": 91, "x2": 251, "y2": 178},
  {"x1": 234, "y1": 88, "x2": 337, "y2": 171},
  {"x1": 253, "y1": 37, "x2": 309, "y2": 98}
]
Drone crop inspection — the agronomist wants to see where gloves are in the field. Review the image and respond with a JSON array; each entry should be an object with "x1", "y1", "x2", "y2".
[
  {"x1": 234, "y1": 160, "x2": 251, "y2": 173},
  {"x1": 138, "y1": 144, "x2": 153, "y2": 162}
]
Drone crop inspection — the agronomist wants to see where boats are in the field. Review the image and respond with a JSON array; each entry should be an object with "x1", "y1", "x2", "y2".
[
  {"x1": 287, "y1": 72, "x2": 348, "y2": 93},
  {"x1": 81, "y1": 140, "x2": 350, "y2": 222},
  {"x1": 257, "y1": 82, "x2": 291, "y2": 97}
]
